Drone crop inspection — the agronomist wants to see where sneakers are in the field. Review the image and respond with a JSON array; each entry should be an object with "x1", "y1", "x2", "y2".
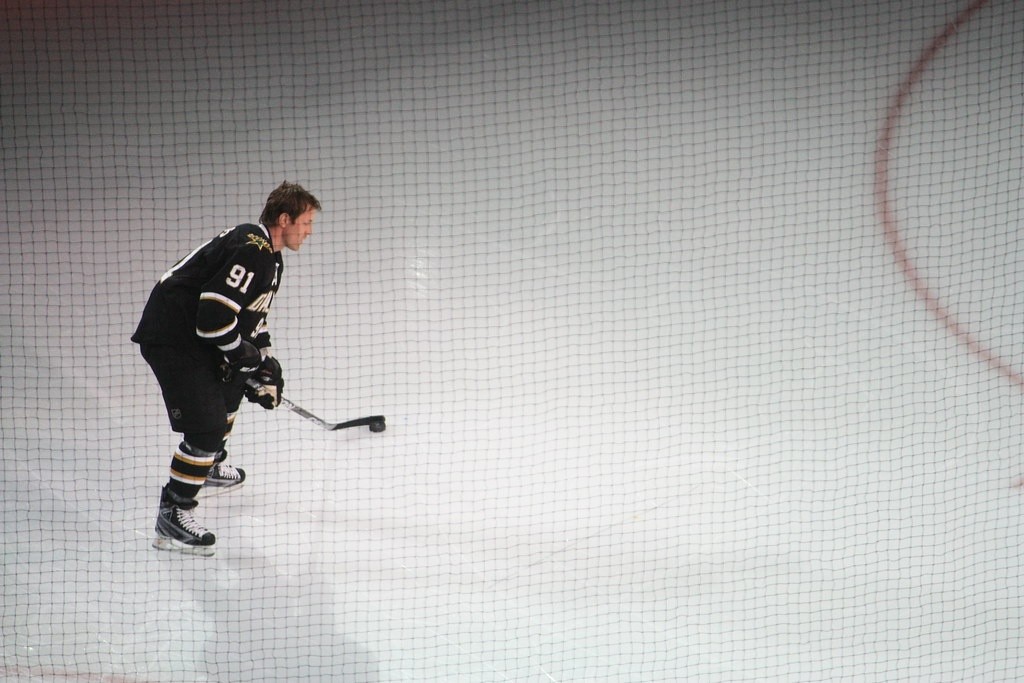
[
  {"x1": 194, "y1": 449, "x2": 246, "y2": 499},
  {"x1": 152, "y1": 483, "x2": 216, "y2": 557}
]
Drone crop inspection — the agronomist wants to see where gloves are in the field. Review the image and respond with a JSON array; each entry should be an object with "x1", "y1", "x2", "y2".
[
  {"x1": 216, "y1": 339, "x2": 261, "y2": 384},
  {"x1": 245, "y1": 355, "x2": 284, "y2": 409}
]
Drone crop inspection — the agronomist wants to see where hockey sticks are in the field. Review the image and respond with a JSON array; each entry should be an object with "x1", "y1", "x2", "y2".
[{"x1": 220, "y1": 361, "x2": 384, "y2": 431}]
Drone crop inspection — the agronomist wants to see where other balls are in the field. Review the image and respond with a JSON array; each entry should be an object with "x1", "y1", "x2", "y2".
[{"x1": 369, "y1": 421, "x2": 386, "y2": 432}]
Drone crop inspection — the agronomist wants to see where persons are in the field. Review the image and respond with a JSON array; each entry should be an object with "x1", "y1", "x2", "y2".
[{"x1": 129, "y1": 182, "x2": 323, "y2": 557}]
